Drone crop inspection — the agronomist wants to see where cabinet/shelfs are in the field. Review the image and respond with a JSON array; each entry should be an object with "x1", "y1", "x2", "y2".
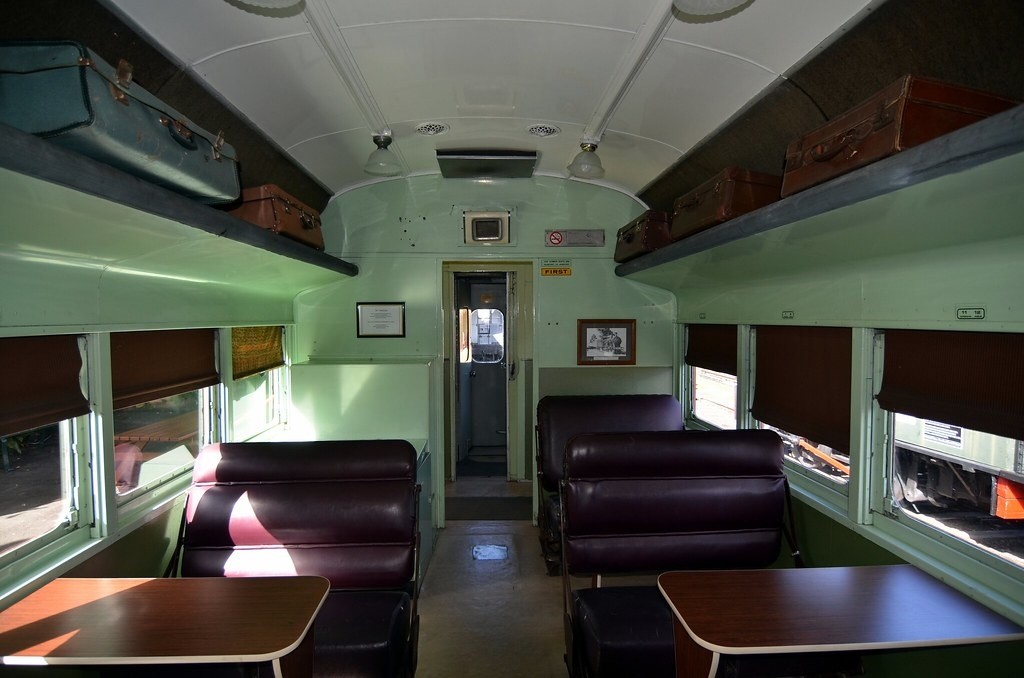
[{"x1": 411, "y1": 440, "x2": 435, "y2": 576}]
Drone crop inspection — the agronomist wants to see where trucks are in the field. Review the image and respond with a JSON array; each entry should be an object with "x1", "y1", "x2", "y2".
[{"x1": 695, "y1": 368, "x2": 1024, "y2": 526}]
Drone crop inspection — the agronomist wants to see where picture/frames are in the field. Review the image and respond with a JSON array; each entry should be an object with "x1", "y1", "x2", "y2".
[{"x1": 576, "y1": 319, "x2": 638, "y2": 365}]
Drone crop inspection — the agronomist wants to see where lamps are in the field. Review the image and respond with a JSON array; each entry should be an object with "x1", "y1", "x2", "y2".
[
  {"x1": 364, "y1": 135, "x2": 402, "y2": 177},
  {"x1": 566, "y1": 143, "x2": 607, "y2": 181}
]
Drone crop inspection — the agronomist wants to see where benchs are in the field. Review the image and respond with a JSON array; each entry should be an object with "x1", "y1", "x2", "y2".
[
  {"x1": 536, "y1": 394, "x2": 686, "y2": 575},
  {"x1": 164, "y1": 440, "x2": 422, "y2": 678},
  {"x1": 559, "y1": 429, "x2": 796, "y2": 678}
]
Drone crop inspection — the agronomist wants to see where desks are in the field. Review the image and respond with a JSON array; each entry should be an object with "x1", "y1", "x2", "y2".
[
  {"x1": 0, "y1": 575, "x2": 331, "y2": 678},
  {"x1": 655, "y1": 564, "x2": 1024, "y2": 678},
  {"x1": 114, "y1": 410, "x2": 198, "y2": 486}
]
